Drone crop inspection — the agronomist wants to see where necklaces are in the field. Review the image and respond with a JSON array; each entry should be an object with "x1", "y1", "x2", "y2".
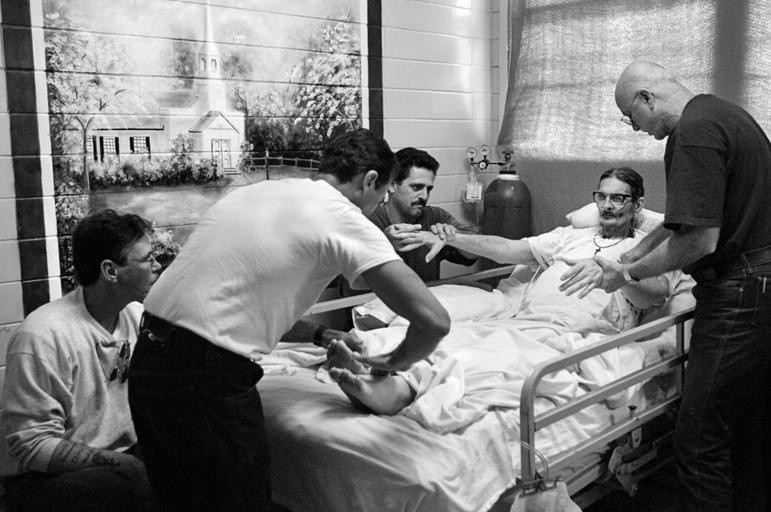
[{"x1": 592, "y1": 222, "x2": 628, "y2": 256}]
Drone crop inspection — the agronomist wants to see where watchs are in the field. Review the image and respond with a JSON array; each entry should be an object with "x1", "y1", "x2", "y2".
[
  {"x1": 311, "y1": 321, "x2": 329, "y2": 349},
  {"x1": 620, "y1": 264, "x2": 642, "y2": 289}
]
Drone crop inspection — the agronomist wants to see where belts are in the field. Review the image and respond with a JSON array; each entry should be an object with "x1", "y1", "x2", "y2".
[{"x1": 689, "y1": 248, "x2": 770, "y2": 282}]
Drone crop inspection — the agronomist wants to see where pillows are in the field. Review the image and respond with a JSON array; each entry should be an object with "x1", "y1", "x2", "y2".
[{"x1": 568, "y1": 203, "x2": 664, "y2": 234}]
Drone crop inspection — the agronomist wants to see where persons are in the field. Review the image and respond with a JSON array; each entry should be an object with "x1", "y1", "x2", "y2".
[
  {"x1": 364, "y1": 145, "x2": 483, "y2": 283},
  {"x1": 125, "y1": 125, "x2": 454, "y2": 512},
  {"x1": 0, "y1": 206, "x2": 165, "y2": 512},
  {"x1": 593, "y1": 58, "x2": 771, "y2": 512},
  {"x1": 324, "y1": 165, "x2": 676, "y2": 420}
]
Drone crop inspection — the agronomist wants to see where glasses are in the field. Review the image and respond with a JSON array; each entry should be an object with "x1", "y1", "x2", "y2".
[
  {"x1": 129, "y1": 245, "x2": 161, "y2": 267},
  {"x1": 592, "y1": 190, "x2": 633, "y2": 205},
  {"x1": 620, "y1": 92, "x2": 639, "y2": 127}
]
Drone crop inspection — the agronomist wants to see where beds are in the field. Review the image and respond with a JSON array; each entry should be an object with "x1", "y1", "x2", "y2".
[{"x1": 258, "y1": 267, "x2": 698, "y2": 512}]
